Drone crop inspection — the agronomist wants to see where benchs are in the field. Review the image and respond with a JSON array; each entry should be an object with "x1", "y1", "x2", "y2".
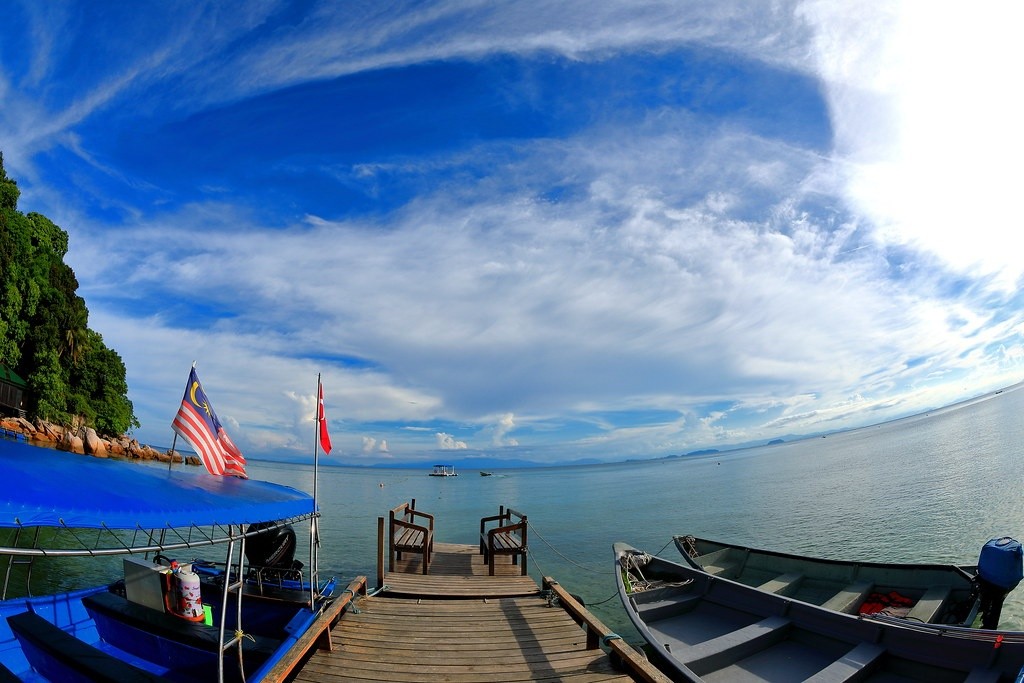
[
  {"x1": 628, "y1": 593, "x2": 703, "y2": 623},
  {"x1": 820, "y1": 581, "x2": 874, "y2": 615},
  {"x1": 6, "y1": 611, "x2": 167, "y2": 683},
  {"x1": 755, "y1": 572, "x2": 805, "y2": 597},
  {"x1": 962, "y1": 667, "x2": 1004, "y2": 683},
  {"x1": 671, "y1": 616, "x2": 793, "y2": 677},
  {"x1": 191, "y1": 560, "x2": 316, "y2": 606},
  {"x1": 800, "y1": 641, "x2": 888, "y2": 683},
  {"x1": 389, "y1": 502, "x2": 434, "y2": 574},
  {"x1": 481, "y1": 508, "x2": 528, "y2": 576},
  {"x1": 82, "y1": 592, "x2": 282, "y2": 680},
  {"x1": 905, "y1": 587, "x2": 952, "y2": 623},
  {"x1": 694, "y1": 559, "x2": 738, "y2": 579}
]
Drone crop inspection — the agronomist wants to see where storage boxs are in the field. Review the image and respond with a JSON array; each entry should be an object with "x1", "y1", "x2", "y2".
[{"x1": 124, "y1": 557, "x2": 171, "y2": 613}]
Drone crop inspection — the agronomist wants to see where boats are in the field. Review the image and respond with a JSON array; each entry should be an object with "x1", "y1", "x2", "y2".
[
  {"x1": 0, "y1": 428, "x2": 338, "y2": 683},
  {"x1": 429, "y1": 465, "x2": 458, "y2": 476},
  {"x1": 613, "y1": 540, "x2": 1024, "y2": 683},
  {"x1": 479, "y1": 471, "x2": 492, "y2": 476},
  {"x1": 672, "y1": 532, "x2": 1024, "y2": 635}
]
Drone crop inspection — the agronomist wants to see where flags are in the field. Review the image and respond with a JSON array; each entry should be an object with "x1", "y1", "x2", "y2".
[
  {"x1": 170, "y1": 365, "x2": 248, "y2": 479},
  {"x1": 319, "y1": 380, "x2": 333, "y2": 455}
]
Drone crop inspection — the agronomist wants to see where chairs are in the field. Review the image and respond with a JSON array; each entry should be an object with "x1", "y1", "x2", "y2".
[{"x1": 165, "y1": 573, "x2": 205, "y2": 624}]
